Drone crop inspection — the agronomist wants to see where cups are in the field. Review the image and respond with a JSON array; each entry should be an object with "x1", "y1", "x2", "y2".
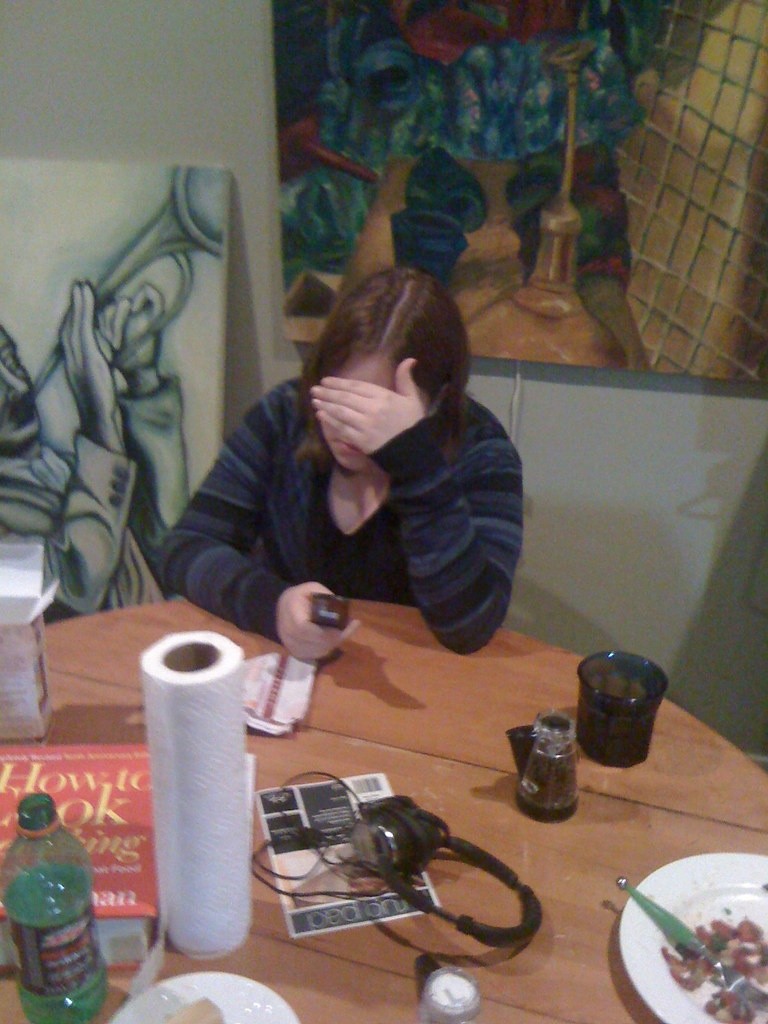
[{"x1": 578, "y1": 652, "x2": 667, "y2": 767}]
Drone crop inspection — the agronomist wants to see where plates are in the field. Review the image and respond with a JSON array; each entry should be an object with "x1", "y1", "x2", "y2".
[
  {"x1": 619, "y1": 853, "x2": 768, "y2": 1024},
  {"x1": 106, "y1": 972, "x2": 302, "y2": 1024}
]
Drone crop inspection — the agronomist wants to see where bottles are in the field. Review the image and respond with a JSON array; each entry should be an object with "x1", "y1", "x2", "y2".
[
  {"x1": 517, "y1": 710, "x2": 578, "y2": 823},
  {"x1": 0, "y1": 794, "x2": 106, "y2": 1024}
]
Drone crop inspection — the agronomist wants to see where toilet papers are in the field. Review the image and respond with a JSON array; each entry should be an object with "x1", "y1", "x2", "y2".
[{"x1": 138, "y1": 633, "x2": 248, "y2": 953}]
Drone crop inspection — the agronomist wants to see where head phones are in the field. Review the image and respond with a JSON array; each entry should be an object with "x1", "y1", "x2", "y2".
[{"x1": 353, "y1": 794, "x2": 543, "y2": 948}]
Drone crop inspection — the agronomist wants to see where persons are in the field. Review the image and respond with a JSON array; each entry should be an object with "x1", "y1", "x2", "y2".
[{"x1": 158, "y1": 266, "x2": 525, "y2": 665}]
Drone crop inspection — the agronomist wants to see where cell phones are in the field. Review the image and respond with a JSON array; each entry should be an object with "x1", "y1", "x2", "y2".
[{"x1": 310, "y1": 594, "x2": 350, "y2": 632}]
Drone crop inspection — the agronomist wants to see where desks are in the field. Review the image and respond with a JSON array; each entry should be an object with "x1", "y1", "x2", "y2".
[{"x1": 0, "y1": 599, "x2": 768, "y2": 1024}]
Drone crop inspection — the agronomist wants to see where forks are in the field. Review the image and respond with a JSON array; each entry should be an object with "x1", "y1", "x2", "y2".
[{"x1": 617, "y1": 878, "x2": 768, "y2": 1012}]
